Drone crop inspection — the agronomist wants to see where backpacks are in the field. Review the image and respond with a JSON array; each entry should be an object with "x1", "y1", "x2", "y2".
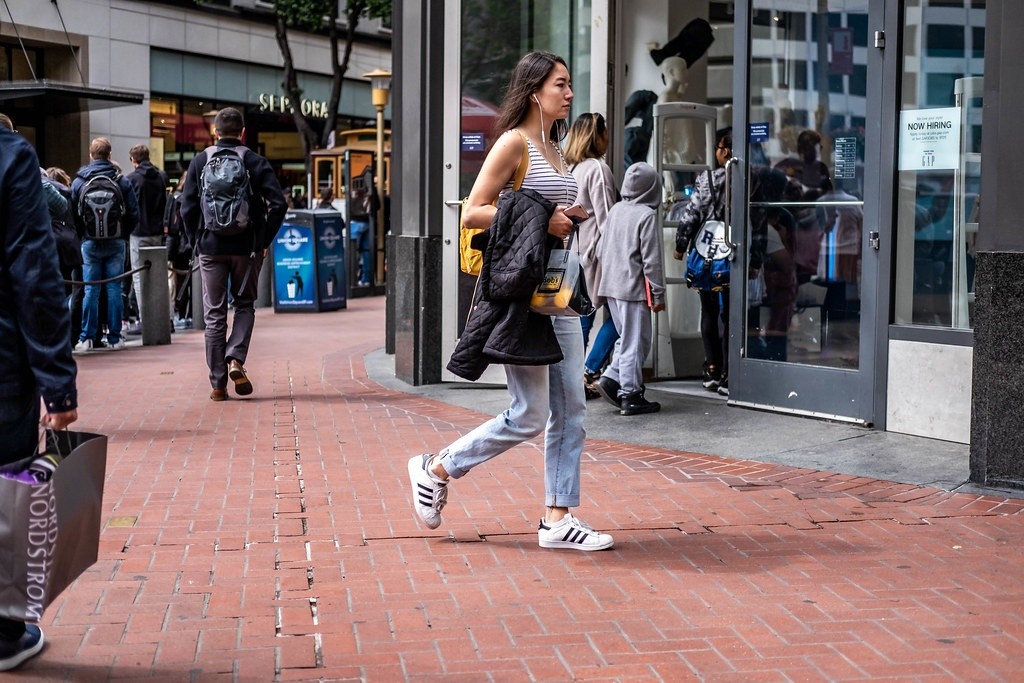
[
  {"x1": 201, "y1": 145, "x2": 258, "y2": 232},
  {"x1": 77, "y1": 173, "x2": 126, "y2": 238},
  {"x1": 685, "y1": 169, "x2": 750, "y2": 293}
]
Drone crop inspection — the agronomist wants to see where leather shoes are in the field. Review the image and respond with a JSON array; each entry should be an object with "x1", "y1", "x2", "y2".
[
  {"x1": 229, "y1": 359, "x2": 253, "y2": 395},
  {"x1": 210, "y1": 387, "x2": 229, "y2": 401}
]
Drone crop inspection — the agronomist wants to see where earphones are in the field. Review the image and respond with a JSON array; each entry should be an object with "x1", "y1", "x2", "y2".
[{"x1": 532, "y1": 93, "x2": 539, "y2": 104}]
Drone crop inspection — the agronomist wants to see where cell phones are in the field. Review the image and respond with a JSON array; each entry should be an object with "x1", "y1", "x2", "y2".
[{"x1": 563, "y1": 203, "x2": 589, "y2": 224}]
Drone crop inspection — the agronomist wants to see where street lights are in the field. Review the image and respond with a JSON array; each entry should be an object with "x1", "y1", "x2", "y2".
[{"x1": 361, "y1": 68, "x2": 394, "y2": 293}]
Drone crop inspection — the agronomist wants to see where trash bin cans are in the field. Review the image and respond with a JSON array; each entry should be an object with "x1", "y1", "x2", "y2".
[{"x1": 270, "y1": 207, "x2": 348, "y2": 312}]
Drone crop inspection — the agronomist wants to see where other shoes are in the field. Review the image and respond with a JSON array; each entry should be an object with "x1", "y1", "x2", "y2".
[
  {"x1": 171, "y1": 320, "x2": 176, "y2": 332},
  {"x1": 126, "y1": 322, "x2": 143, "y2": 335},
  {"x1": 93, "y1": 341, "x2": 107, "y2": 349},
  {"x1": 0, "y1": 624, "x2": 44, "y2": 670},
  {"x1": 174, "y1": 318, "x2": 192, "y2": 330},
  {"x1": 584, "y1": 370, "x2": 601, "y2": 392}
]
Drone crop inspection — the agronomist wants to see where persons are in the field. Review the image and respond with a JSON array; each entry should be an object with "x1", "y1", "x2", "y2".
[
  {"x1": 675, "y1": 134, "x2": 768, "y2": 395},
  {"x1": 317, "y1": 187, "x2": 346, "y2": 230},
  {"x1": 0, "y1": 122, "x2": 78, "y2": 463},
  {"x1": 284, "y1": 186, "x2": 308, "y2": 209},
  {"x1": 598, "y1": 162, "x2": 666, "y2": 415},
  {"x1": 40, "y1": 138, "x2": 168, "y2": 351},
  {"x1": 564, "y1": 113, "x2": 616, "y2": 395},
  {"x1": 351, "y1": 187, "x2": 390, "y2": 286},
  {"x1": 762, "y1": 129, "x2": 862, "y2": 359},
  {"x1": 408, "y1": 51, "x2": 612, "y2": 551},
  {"x1": 647, "y1": 57, "x2": 697, "y2": 194},
  {"x1": 166, "y1": 108, "x2": 289, "y2": 401}
]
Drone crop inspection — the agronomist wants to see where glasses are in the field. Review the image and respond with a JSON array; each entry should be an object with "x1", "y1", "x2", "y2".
[{"x1": 713, "y1": 145, "x2": 726, "y2": 151}]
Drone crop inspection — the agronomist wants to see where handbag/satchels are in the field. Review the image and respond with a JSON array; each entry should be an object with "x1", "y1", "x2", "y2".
[
  {"x1": 457, "y1": 193, "x2": 499, "y2": 276},
  {"x1": 51, "y1": 220, "x2": 84, "y2": 267},
  {"x1": 530, "y1": 226, "x2": 596, "y2": 318},
  {"x1": 0, "y1": 423, "x2": 108, "y2": 623}
]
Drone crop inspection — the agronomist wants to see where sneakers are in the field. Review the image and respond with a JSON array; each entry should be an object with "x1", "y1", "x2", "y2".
[
  {"x1": 408, "y1": 453, "x2": 449, "y2": 529},
  {"x1": 538, "y1": 514, "x2": 614, "y2": 550},
  {"x1": 74, "y1": 338, "x2": 93, "y2": 350},
  {"x1": 716, "y1": 370, "x2": 729, "y2": 396},
  {"x1": 594, "y1": 376, "x2": 619, "y2": 407},
  {"x1": 701, "y1": 365, "x2": 723, "y2": 387},
  {"x1": 107, "y1": 337, "x2": 125, "y2": 350},
  {"x1": 620, "y1": 393, "x2": 661, "y2": 415}
]
